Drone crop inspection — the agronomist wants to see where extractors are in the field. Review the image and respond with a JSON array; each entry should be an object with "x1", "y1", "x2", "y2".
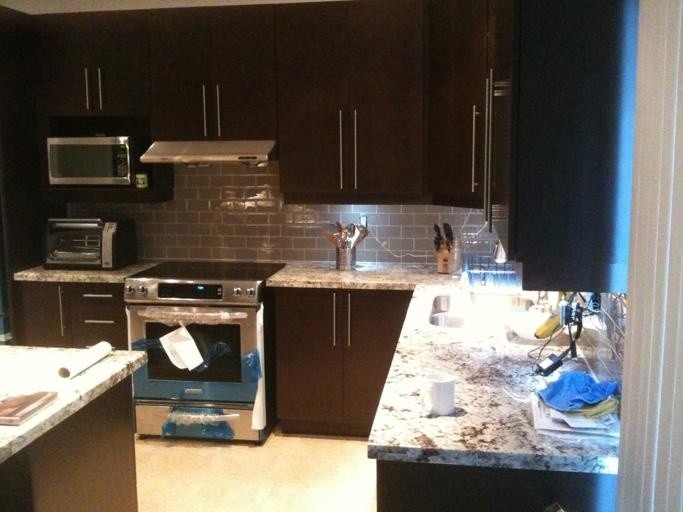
[{"x1": 138, "y1": 139, "x2": 279, "y2": 168}]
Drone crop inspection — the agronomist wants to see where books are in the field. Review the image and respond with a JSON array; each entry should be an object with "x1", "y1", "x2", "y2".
[{"x1": 0, "y1": 387, "x2": 59, "y2": 427}]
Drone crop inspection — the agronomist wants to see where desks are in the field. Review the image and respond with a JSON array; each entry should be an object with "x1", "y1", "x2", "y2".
[{"x1": 1, "y1": 342, "x2": 149, "y2": 511}]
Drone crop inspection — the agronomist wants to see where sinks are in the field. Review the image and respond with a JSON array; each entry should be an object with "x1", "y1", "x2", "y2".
[
  {"x1": 428, "y1": 311, "x2": 502, "y2": 330},
  {"x1": 428, "y1": 293, "x2": 535, "y2": 315}
]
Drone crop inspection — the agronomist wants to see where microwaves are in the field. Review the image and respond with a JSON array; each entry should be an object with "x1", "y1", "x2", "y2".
[{"x1": 45, "y1": 136, "x2": 140, "y2": 186}]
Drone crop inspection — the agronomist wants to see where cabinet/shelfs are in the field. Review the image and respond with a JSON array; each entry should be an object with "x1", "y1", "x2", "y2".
[
  {"x1": 20, "y1": 280, "x2": 71, "y2": 348},
  {"x1": 146, "y1": 4, "x2": 277, "y2": 141},
  {"x1": 274, "y1": 285, "x2": 415, "y2": 439},
  {"x1": 428, "y1": 1, "x2": 496, "y2": 208},
  {"x1": 69, "y1": 282, "x2": 129, "y2": 352},
  {"x1": 274, "y1": 0, "x2": 434, "y2": 203},
  {"x1": 484, "y1": 1, "x2": 628, "y2": 295},
  {"x1": 34, "y1": 8, "x2": 175, "y2": 203}
]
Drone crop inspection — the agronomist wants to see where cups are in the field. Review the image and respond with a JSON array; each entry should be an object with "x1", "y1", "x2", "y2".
[
  {"x1": 135, "y1": 174, "x2": 148, "y2": 189},
  {"x1": 335, "y1": 246, "x2": 357, "y2": 271},
  {"x1": 423, "y1": 372, "x2": 457, "y2": 417}
]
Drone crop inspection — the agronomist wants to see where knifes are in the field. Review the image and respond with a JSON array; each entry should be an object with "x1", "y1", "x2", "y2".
[{"x1": 433, "y1": 222, "x2": 453, "y2": 253}]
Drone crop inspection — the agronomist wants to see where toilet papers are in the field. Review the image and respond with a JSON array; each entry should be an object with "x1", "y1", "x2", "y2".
[{"x1": 55, "y1": 342, "x2": 112, "y2": 380}]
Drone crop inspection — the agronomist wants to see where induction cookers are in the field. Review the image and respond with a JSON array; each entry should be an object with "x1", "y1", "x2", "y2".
[{"x1": 123, "y1": 260, "x2": 287, "y2": 305}]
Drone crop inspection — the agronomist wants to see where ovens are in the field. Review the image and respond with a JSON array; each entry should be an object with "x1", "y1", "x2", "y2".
[
  {"x1": 38, "y1": 216, "x2": 138, "y2": 270},
  {"x1": 125, "y1": 304, "x2": 277, "y2": 446}
]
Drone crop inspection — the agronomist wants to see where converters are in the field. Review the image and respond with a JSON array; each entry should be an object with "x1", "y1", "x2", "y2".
[{"x1": 535, "y1": 353, "x2": 563, "y2": 376}]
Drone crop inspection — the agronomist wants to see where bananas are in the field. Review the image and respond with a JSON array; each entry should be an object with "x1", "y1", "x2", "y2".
[{"x1": 534, "y1": 291, "x2": 566, "y2": 339}]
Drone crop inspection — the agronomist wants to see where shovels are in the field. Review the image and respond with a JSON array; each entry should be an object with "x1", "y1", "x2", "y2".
[{"x1": 325, "y1": 223, "x2": 346, "y2": 248}]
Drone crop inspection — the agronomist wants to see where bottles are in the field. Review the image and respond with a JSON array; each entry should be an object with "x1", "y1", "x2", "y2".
[{"x1": 447, "y1": 239, "x2": 465, "y2": 280}]
